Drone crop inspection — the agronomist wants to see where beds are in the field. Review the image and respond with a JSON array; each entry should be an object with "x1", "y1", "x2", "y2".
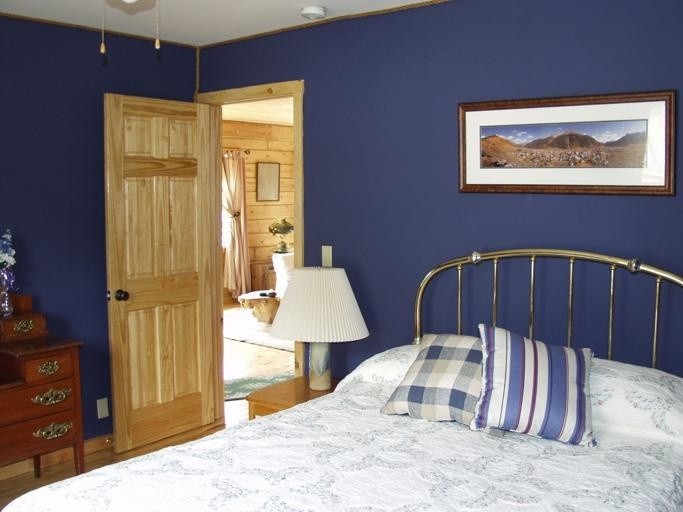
[{"x1": 0, "y1": 248, "x2": 683, "y2": 512}]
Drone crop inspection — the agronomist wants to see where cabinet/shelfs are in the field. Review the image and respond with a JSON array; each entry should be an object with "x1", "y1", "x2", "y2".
[{"x1": 0, "y1": 294, "x2": 85, "y2": 478}]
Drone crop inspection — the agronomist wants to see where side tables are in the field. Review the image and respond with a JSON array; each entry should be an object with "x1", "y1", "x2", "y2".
[{"x1": 246, "y1": 376, "x2": 342, "y2": 421}]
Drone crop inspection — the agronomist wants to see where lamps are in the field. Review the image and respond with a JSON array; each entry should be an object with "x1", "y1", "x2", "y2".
[{"x1": 269, "y1": 267, "x2": 370, "y2": 391}]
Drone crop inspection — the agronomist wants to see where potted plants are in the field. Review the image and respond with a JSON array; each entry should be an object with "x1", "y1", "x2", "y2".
[{"x1": 268, "y1": 218, "x2": 294, "y2": 253}]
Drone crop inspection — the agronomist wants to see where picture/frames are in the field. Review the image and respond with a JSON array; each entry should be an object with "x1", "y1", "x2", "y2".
[
  {"x1": 256, "y1": 162, "x2": 280, "y2": 201},
  {"x1": 457, "y1": 90, "x2": 676, "y2": 196}
]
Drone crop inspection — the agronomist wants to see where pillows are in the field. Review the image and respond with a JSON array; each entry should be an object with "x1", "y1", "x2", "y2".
[
  {"x1": 588, "y1": 357, "x2": 682, "y2": 447},
  {"x1": 335, "y1": 343, "x2": 421, "y2": 412},
  {"x1": 381, "y1": 333, "x2": 505, "y2": 439},
  {"x1": 468, "y1": 323, "x2": 599, "y2": 449}
]
